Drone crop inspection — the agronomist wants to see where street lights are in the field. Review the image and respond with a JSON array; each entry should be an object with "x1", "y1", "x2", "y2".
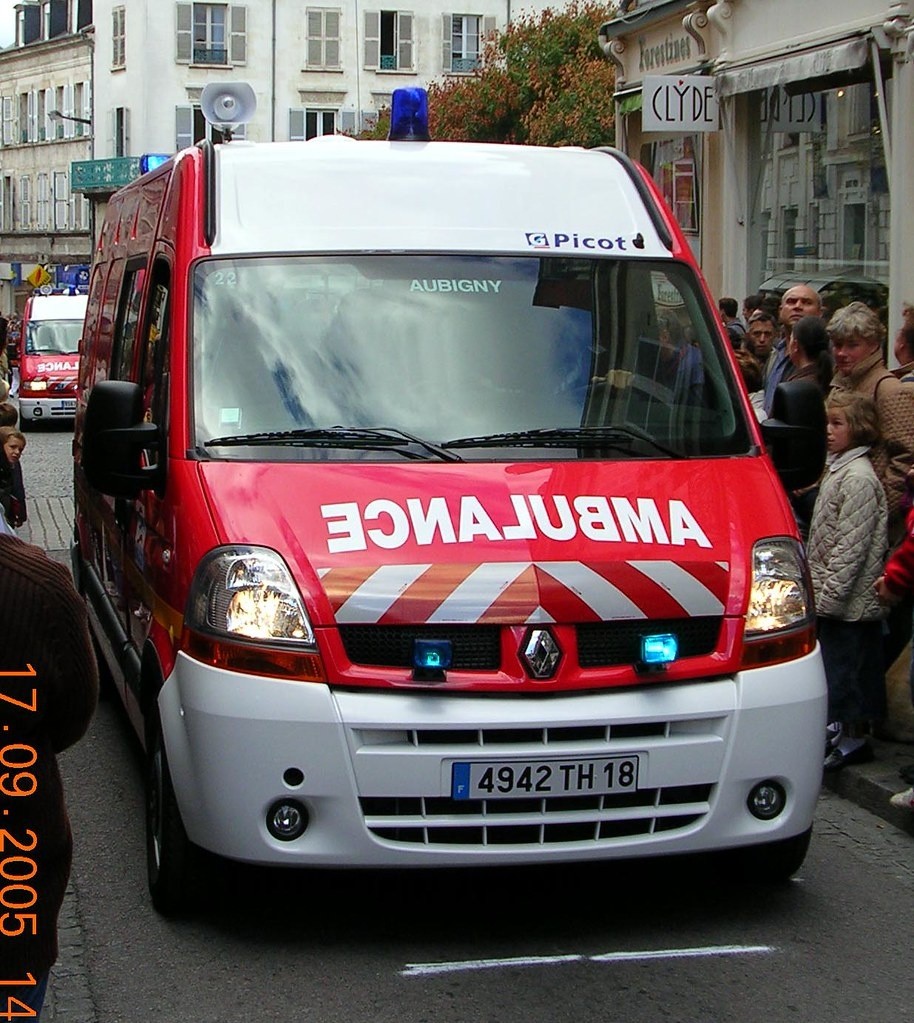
[{"x1": 47, "y1": 112, "x2": 96, "y2": 266}]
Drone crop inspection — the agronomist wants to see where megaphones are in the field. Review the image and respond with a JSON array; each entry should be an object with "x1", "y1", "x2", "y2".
[
  {"x1": 40, "y1": 285, "x2": 52, "y2": 294},
  {"x1": 200, "y1": 82, "x2": 257, "y2": 132}
]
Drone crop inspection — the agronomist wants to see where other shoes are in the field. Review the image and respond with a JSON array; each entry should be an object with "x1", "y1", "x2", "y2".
[
  {"x1": 824, "y1": 743, "x2": 874, "y2": 769},
  {"x1": 899, "y1": 764, "x2": 914, "y2": 780},
  {"x1": 889, "y1": 787, "x2": 914, "y2": 808}
]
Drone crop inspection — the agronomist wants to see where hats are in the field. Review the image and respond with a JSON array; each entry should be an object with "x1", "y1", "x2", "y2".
[{"x1": 747, "y1": 308, "x2": 763, "y2": 324}]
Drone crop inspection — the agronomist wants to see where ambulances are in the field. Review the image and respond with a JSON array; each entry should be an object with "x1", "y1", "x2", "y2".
[
  {"x1": 69, "y1": 79, "x2": 826, "y2": 922},
  {"x1": 7, "y1": 286, "x2": 91, "y2": 432}
]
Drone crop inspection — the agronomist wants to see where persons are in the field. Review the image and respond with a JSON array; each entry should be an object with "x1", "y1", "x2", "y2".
[
  {"x1": 1, "y1": 532, "x2": 100, "y2": 1023},
  {"x1": 0, "y1": 307, "x2": 27, "y2": 541},
  {"x1": 652, "y1": 284, "x2": 914, "y2": 806}
]
